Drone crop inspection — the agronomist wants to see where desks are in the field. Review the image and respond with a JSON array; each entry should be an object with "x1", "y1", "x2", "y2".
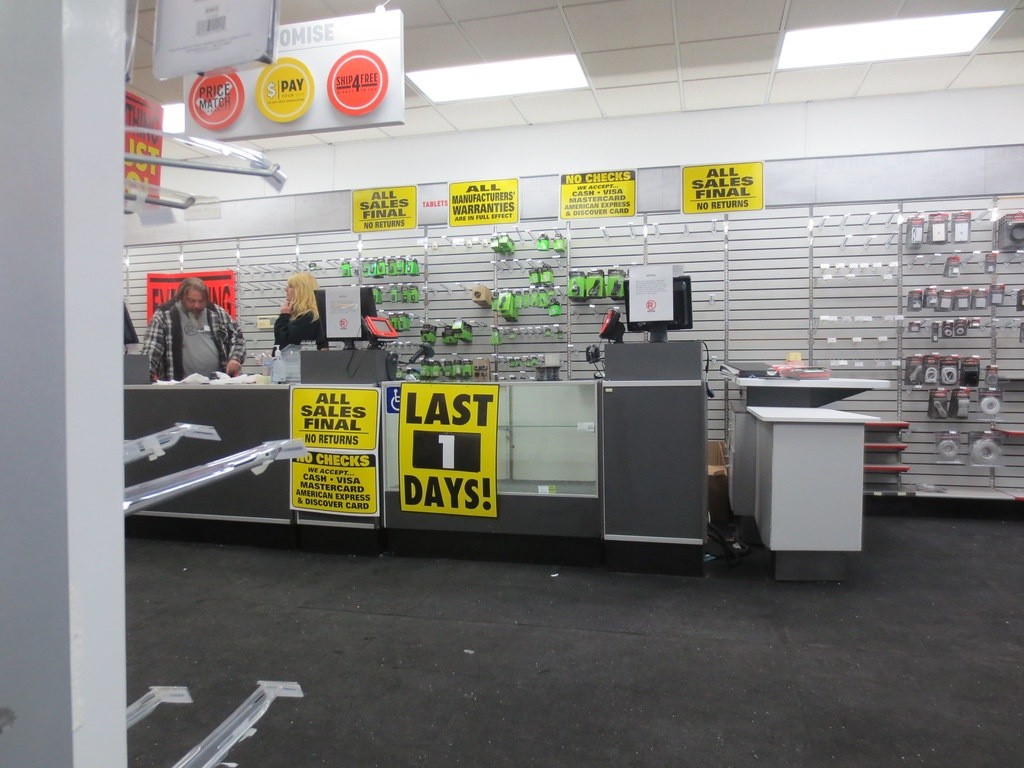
[{"x1": 746, "y1": 407, "x2": 880, "y2": 580}]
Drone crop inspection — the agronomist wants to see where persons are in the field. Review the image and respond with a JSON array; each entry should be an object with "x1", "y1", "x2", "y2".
[
  {"x1": 272, "y1": 272, "x2": 320, "y2": 357},
  {"x1": 142, "y1": 277, "x2": 246, "y2": 385}
]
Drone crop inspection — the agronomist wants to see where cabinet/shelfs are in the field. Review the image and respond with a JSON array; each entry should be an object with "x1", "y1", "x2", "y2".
[
  {"x1": 863, "y1": 421, "x2": 911, "y2": 472},
  {"x1": 728, "y1": 371, "x2": 891, "y2": 515}
]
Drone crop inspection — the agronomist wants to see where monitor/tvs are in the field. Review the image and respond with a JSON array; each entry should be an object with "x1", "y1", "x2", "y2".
[
  {"x1": 623, "y1": 275, "x2": 692, "y2": 343},
  {"x1": 314, "y1": 287, "x2": 378, "y2": 350}
]
